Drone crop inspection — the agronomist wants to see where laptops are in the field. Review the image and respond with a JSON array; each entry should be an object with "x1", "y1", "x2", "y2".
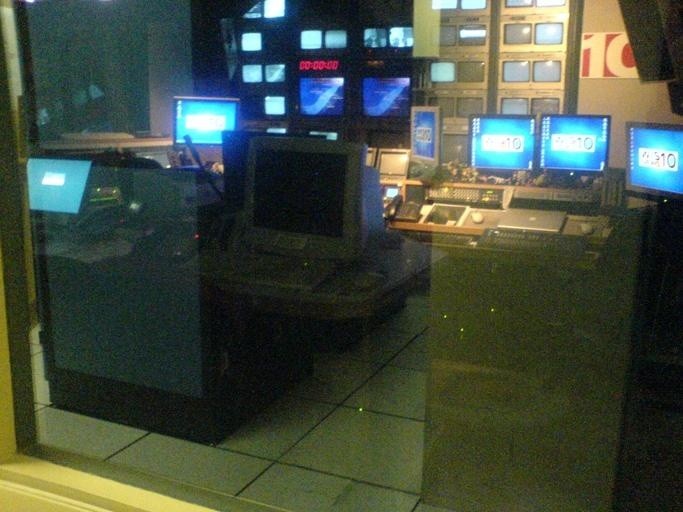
[{"x1": 501, "y1": 207, "x2": 568, "y2": 233}]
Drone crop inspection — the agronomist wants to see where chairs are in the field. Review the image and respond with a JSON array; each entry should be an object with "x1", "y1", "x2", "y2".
[{"x1": 33, "y1": 254, "x2": 298, "y2": 446}]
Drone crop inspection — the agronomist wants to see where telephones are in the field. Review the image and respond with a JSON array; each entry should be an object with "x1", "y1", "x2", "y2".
[{"x1": 386, "y1": 194, "x2": 424, "y2": 223}]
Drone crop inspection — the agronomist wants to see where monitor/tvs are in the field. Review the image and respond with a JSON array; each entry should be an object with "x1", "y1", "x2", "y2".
[
  {"x1": 173, "y1": 95, "x2": 241, "y2": 146},
  {"x1": 376, "y1": 148, "x2": 412, "y2": 184},
  {"x1": 241, "y1": 0, "x2": 413, "y2": 148},
  {"x1": 410, "y1": 105, "x2": 442, "y2": 168},
  {"x1": 365, "y1": 146, "x2": 377, "y2": 167},
  {"x1": 239, "y1": 135, "x2": 365, "y2": 261},
  {"x1": 624, "y1": 121, "x2": 683, "y2": 203},
  {"x1": 468, "y1": 114, "x2": 537, "y2": 179},
  {"x1": 425, "y1": 0, "x2": 570, "y2": 119},
  {"x1": 538, "y1": 113, "x2": 610, "y2": 178},
  {"x1": 22, "y1": 156, "x2": 91, "y2": 217}
]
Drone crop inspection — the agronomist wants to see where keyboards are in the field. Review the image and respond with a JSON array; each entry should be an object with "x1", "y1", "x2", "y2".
[
  {"x1": 207, "y1": 255, "x2": 334, "y2": 292},
  {"x1": 393, "y1": 231, "x2": 472, "y2": 245},
  {"x1": 477, "y1": 226, "x2": 589, "y2": 256}
]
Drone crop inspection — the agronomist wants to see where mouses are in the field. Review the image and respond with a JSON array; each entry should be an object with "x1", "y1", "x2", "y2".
[
  {"x1": 579, "y1": 219, "x2": 593, "y2": 236},
  {"x1": 471, "y1": 211, "x2": 485, "y2": 224}
]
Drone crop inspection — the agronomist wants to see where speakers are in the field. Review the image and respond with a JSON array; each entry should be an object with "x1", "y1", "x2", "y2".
[
  {"x1": 618, "y1": 0, "x2": 683, "y2": 81},
  {"x1": 667, "y1": 78, "x2": 683, "y2": 117}
]
